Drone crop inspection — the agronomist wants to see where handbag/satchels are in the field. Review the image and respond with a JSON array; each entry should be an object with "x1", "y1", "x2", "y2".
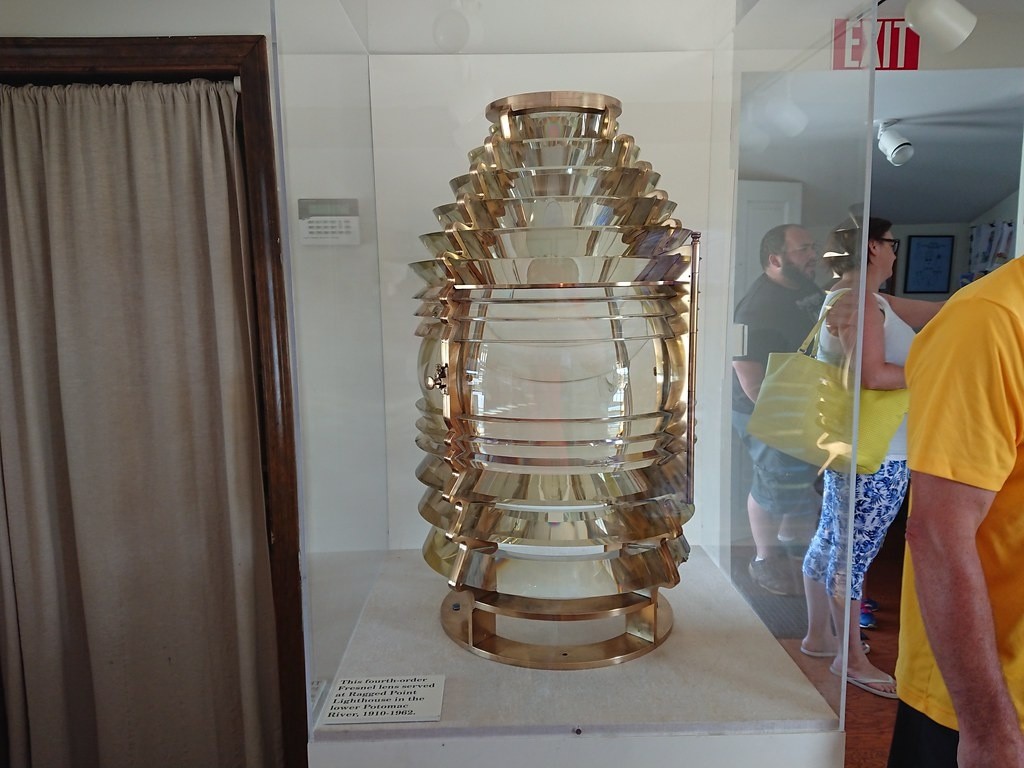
[{"x1": 745, "y1": 288, "x2": 911, "y2": 476}]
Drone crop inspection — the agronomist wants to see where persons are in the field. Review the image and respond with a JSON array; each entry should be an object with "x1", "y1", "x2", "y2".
[
  {"x1": 886, "y1": 254, "x2": 1024, "y2": 768},
  {"x1": 801, "y1": 215, "x2": 948, "y2": 698},
  {"x1": 732, "y1": 223, "x2": 826, "y2": 597}
]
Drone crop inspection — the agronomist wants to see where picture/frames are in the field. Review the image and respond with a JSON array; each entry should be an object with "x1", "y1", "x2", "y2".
[
  {"x1": 903, "y1": 235, "x2": 955, "y2": 293},
  {"x1": 879, "y1": 250, "x2": 897, "y2": 296}
]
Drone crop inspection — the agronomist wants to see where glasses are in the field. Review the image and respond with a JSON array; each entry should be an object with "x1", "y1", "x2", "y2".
[{"x1": 875, "y1": 237, "x2": 901, "y2": 253}]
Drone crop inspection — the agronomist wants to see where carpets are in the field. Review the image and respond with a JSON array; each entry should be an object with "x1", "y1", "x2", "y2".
[{"x1": 730, "y1": 544, "x2": 870, "y2": 642}]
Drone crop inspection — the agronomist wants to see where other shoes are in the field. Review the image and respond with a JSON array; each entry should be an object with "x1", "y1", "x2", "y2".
[
  {"x1": 786, "y1": 543, "x2": 810, "y2": 559},
  {"x1": 748, "y1": 554, "x2": 796, "y2": 595}
]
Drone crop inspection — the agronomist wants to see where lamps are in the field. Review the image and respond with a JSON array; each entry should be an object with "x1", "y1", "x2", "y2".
[
  {"x1": 904, "y1": 0, "x2": 977, "y2": 53},
  {"x1": 872, "y1": 117, "x2": 915, "y2": 167}
]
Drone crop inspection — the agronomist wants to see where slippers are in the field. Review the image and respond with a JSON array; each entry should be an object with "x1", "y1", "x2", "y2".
[
  {"x1": 800, "y1": 635, "x2": 870, "y2": 657},
  {"x1": 830, "y1": 658, "x2": 899, "y2": 698}
]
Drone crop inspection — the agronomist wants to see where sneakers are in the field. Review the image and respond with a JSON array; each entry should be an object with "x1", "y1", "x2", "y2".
[
  {"x1": 859, "y1": 599, "x2": 878, "y2": 628},
  {"x1": 860, "y1": 597, "x2": 881, "y2": 611}
]
